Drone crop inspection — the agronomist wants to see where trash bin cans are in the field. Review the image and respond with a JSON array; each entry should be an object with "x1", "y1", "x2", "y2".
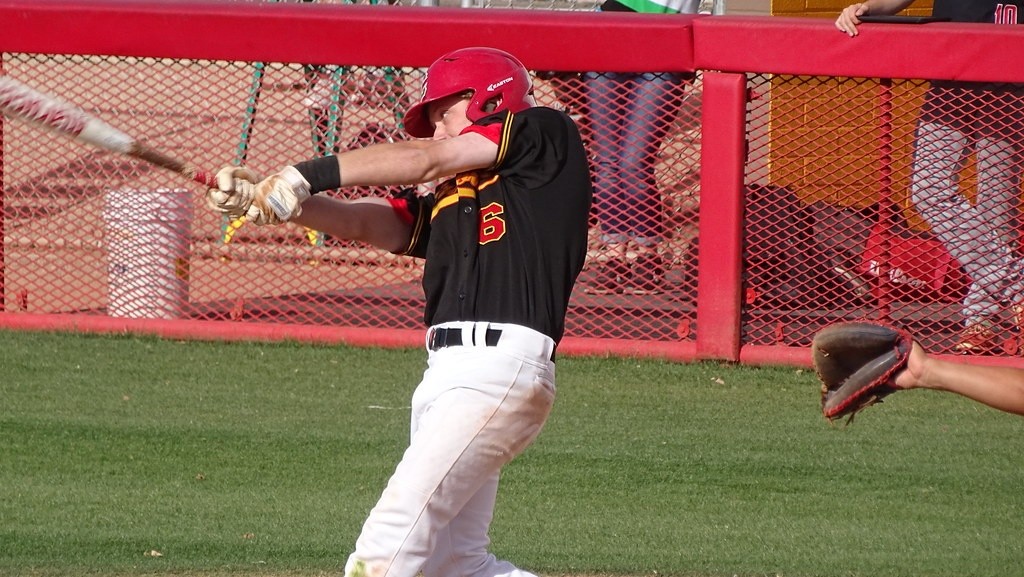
[{"x1": 102, "y1": 187, "x2": 193, "y2": 320}]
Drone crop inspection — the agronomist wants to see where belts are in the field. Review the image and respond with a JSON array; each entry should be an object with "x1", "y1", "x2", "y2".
[{"x1": 430, "y1": 328, "x2": 557, "y2": 362}]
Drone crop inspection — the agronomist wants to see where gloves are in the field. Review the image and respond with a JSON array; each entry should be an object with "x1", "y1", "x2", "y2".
[
  {"x1": 246, "y1": 165, "x2": 312, "y2": 226},
  {"x1": 206, "y1": 165, "x2": 266, "y2": 214}
]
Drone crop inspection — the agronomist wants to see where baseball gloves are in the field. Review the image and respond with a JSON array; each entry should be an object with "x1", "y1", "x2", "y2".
[{"x1": 809, "y1": 322, "x2": 913, "y2": 422}]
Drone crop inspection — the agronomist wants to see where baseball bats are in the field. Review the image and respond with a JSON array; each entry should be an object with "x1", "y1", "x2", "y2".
[{"x1": 0, "y1": 73, "x2": 218, "y2": 190}]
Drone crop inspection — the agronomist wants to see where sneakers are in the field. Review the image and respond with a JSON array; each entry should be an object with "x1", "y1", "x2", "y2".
[
  {"x1": 1013, "y1": 305, "x2": 1024, "y2": 327},
  {"x1": 956, "y1": 324, "x2": 997, "y2": 350}
]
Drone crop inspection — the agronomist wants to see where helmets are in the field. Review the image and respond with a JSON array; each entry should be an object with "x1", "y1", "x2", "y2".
[{"x1": 404, "y1": 47, "x2": 539, "y2": 138}]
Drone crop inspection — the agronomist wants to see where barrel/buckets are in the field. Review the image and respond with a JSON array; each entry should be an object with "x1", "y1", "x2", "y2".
[{"x1": 107, "y1": 187, "x2": 191, "y2": 318}]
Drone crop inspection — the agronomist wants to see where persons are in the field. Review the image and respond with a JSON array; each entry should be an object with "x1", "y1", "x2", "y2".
[
  {"x1": 204, "y1": 47, "x2": 592, "y2": 577},
  {"x1": 587, "y1": 0, "x2": 699, "y2": 293},
  {"x1": 813, "y1": 321, "x2": 1024, "y2": 419},
  {"x1": 836, "y1": 1, "x2": 1024, "y2": 352}
]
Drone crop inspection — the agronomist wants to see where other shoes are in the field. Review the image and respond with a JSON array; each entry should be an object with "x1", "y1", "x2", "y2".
[
  {"x1": 624, "y1": 253, "x2": 666, "y2": 294},
  {"x1": 585, "y1": 257, "x2": 630, "y2": 295}
]
